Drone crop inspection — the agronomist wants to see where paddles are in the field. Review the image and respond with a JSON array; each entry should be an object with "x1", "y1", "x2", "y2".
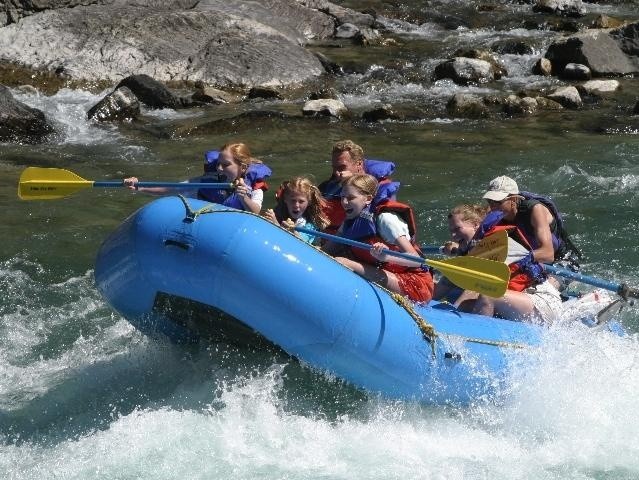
[
  {"x1": 17, "y1": 167, "x2": 235, "y2": 201},
  {"x1": 433, "y1": 229, "x2": 508, "y2": 269},
  {"x1": 287, "y1": 218, "x2": 510, "y2": 298}
]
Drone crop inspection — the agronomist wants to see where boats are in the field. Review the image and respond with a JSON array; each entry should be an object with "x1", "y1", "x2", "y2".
[{"x1": 92, "y1": 196, "x2": 626, "y2": 408}]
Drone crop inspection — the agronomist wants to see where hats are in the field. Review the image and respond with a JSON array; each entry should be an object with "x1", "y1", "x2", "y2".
[{"x1": 481, "y1": 175, "x2": 520, "y2": 201}]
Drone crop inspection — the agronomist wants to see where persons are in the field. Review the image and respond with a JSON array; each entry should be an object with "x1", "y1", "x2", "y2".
[
  {"x1": 316, "y1": 140, "x2": 400, "y2": 245},
  {"x1": 321, "y1": 172, "x2": 435, "y2": 302},
  {"x1": 441, "y1": 203, "x2": 567, "y2": 325},
  {"x1": 122, "y1": 140, "x2": 273, "y2": 219},
  {"x1": 483, "y1": 174, "x2": 577, "y2": 291},
  {"x1": 269, "y1": 173, "x2": 326, "y2": 257}
]
